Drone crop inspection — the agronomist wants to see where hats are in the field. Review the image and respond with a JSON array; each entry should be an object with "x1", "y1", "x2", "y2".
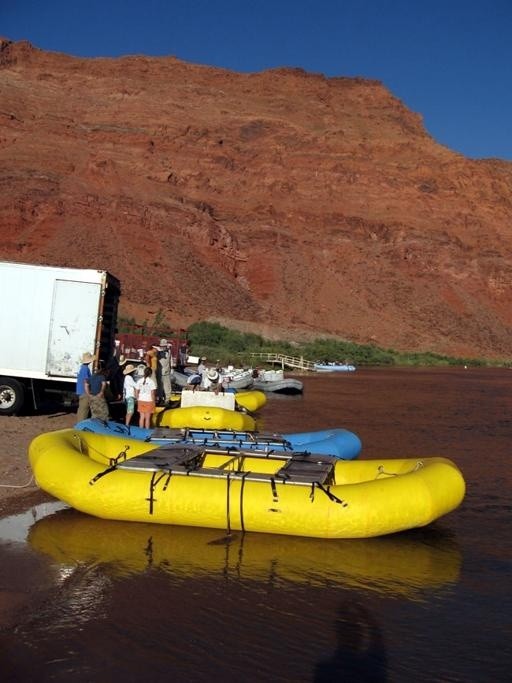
[
  {"x1": 123, "y1": 364, "x2": 136, "y2": 375},
  {"x1": 207, "y1": 370, "x2": 218, "y2": 380},
  {"x1": 119, "y1": 355, "x2": 127, "y2": 366},
  {"x1": 82, "y1": 352, "x2": 96, "y2": 364},
  {"x1": 151, "y1": 339, "x2": 167, "y2": 351},
  {"x1": 201, "y1": 355, "x2": 207, "y2": 361}
]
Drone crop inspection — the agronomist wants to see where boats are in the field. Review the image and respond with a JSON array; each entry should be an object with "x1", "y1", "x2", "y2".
[
  {"x1": 314, "y1": 361, "x2": 356, "y2": 372},
  {"x1": 152, "y1": 367, "x2": 305, "y2": 430}
]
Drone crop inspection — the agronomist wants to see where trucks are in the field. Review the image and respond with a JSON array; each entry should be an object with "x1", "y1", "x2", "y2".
[{"x1": 0, "y1": 261, "x2": 121, "y2": 414}]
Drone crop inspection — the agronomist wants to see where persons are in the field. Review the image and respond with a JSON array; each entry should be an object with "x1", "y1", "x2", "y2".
[
  {"x1": 202, "y1": 370, "x2": 223, "y2": 395},
  {"x1": 75, "y1": 338, "x2": 192, "y2": 430},
  {"x1": 197, "y1": 356, "x2": 208, "y2": 376},
  {"x1": 215, "y1": 359, "x2": 221, "y2": 374},
  {"x1": 228, "y1": 362, "x2": 232, "y2": 372}
]
